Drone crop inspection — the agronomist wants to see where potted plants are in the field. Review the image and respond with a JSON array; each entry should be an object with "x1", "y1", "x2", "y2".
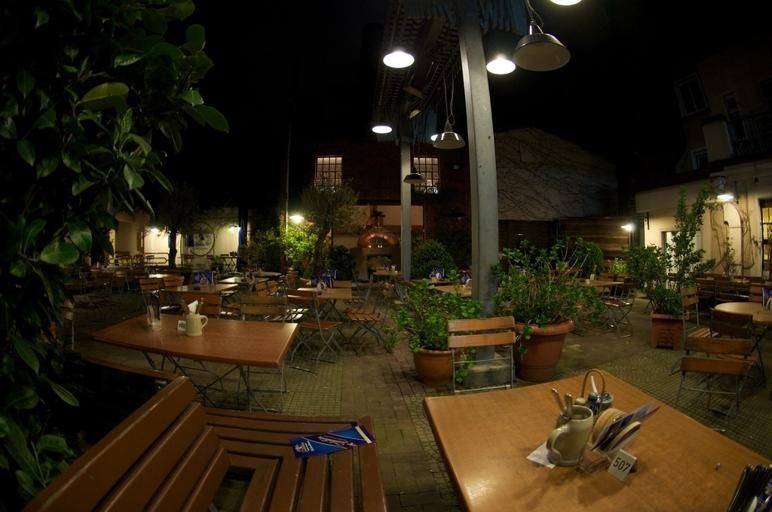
[
  {"x1": 625, "y1": 177, "x2": 717, "y2": 349},
  {"x1": 384, "y1": 276, "x2": 482, "y2": 388},
  {"x1": 484, "y1": 235, "x2": 604, "y2": 384}
]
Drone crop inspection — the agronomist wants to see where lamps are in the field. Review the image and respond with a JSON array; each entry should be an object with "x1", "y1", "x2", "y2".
[
  {"x1": 482, "y1": 28, "x2": 517, "y2": 74},
  {"x1": 432, "y1": 70, "x2": 465, "y2": 150},
  {"x1": 382, "y1": 20, "x2": 416, "y2": 68},
  {"x1": 403, "y1": 123, "x2": 426, "y2": 186},
  {"x1": 371, "y1": 105, "x2": 392, "y2": 135},
  {"x1": 514, "y1": 1, "x2": 572, "y2": 72}
]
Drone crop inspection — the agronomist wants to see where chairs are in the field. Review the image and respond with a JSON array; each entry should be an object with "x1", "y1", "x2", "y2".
[
  {"x1": 40, "y1": 241, "x2": 645, "y2": 374},
  {"x1": 446, "y1": 309, "x2": 517, "y2": 398},
  {"x1": 673, "y1": 269, "x2": 771, "y2": 434}
]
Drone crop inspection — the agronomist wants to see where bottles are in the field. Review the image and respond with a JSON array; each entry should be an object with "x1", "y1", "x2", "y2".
[
  {"x1": 576, "y1": 393, "x2": 599, "y2": 414},
  {"x1": 327, "y1": 274, "x2": 333, "y2": 289}
]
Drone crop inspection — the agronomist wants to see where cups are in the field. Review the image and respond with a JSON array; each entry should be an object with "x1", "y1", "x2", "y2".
[
  {"x1": 545, "y1": 404, "x2": 593, "y2": 465},
  {"x1": 435, "y1": 273, "x2": 441, "y2": 280},
  {"x1": 201, "y1": 278, "x2": 210, "y2": 291},
  {"x1": 183, "y1": 313, "x2": 208, "y2": 337},
  {"x1": 762, "y1": 287, "x2": 772, "y2": 311},
  {"x1": 146, "y1": 305, "x2": 161, "y2": 330},
  {"x1": 595, "y1": 392, "x2": 613, "y2": 410}
]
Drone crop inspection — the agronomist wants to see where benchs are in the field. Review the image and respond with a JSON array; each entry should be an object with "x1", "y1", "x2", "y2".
[{"x1": 23, "y1": 350, "x2": 390, "y2": 511}]
